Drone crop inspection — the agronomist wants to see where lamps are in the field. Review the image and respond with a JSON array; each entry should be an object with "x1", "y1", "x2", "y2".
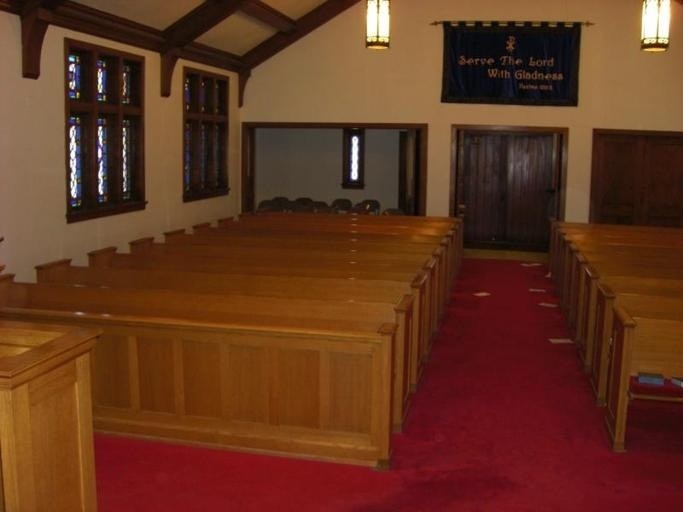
[
  {"x1": 639, "y1": 1, "x2": 671, "y2": 54},
  {"x1": 364, "y1": 0, "x2": 390, "y2": 49}
]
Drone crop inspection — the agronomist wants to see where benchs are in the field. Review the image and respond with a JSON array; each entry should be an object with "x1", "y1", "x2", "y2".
[
  {"x1": 549, "y1": 221, "x2": 682, "y2": 454},
  {"x1": 0, "y1": 205, "x2": 467, "y2": 475}
]
